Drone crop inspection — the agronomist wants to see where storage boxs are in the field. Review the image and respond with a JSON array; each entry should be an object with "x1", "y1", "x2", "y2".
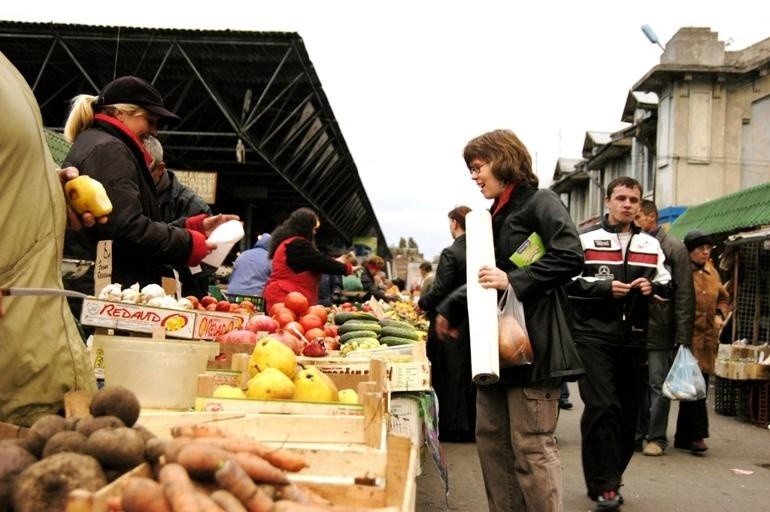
[
  {"x1": 714, "y1": 342, "x2": 770, "y2": 379},
  {"x1": 88, "y1": 332, "x2": 221, "y2": 411}
]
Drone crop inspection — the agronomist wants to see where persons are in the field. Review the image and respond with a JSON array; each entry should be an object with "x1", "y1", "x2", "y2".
[
  {"x1": 342, "y1": 255, "x2": 395, "y2": 301},
  {"x1": 671, "y1": 229, "x2": 734, "y2": 449},
  {"x1": 262, "y1": 208, "x2": 363, "y2": 316},
  {"x1": 629, "y1": 202, "x2": 695, "y2": 457},
  {"x1": 437, "y1": 124, "x2": 587, "y2": 509},
  {"x1": 419, "y1": 261, "x2": 436, "y2": 298},
  {"x1": 227, "y1": 233, "x2": 274, "y2": 298},
  {"x1": 386, "y1": 276, "x2": 406, "y2": 297},
  {"x1": 138, "y1": 131, "x2": 216, "y2": 303},
  {"x1": 557, "y1": 176, "x2": 670, "y2": 509},
  {"x1": 414, "y1": 206, "x2": 477, "y2": 445},
  {"x1": 55, "y1": 166, "x2": 108, "y2": 230},
  {"x1": 1, "y1": 45, "x2": 102, "y2": 425},
  {"x1": 67, "y1": 74, "x2": 244, "y2": 302}
]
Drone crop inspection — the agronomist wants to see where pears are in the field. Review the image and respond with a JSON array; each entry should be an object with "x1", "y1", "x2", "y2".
[
  {"x1": 64, "y1": 174, "x2": 113, "y2": 218},
  {"x1": 211, "y1": 337, "x2": 359, "y2": 406}
]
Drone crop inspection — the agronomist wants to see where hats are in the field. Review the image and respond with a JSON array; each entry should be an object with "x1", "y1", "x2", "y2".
[
  {"x1": 97, "y1": 76, "x2": 181, "y2": 121},
  {"x1": 683, "y1": 231, "x2": 711, "y2": 253}
]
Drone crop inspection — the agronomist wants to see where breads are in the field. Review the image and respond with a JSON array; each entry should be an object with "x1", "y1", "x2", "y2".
[{"x1": 499, "y1": 315, "x2": 535, "y2": 366}]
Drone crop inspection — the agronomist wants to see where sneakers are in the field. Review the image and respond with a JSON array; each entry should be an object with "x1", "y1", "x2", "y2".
[
  {"x1": 674, "y1": 438, "x2": 708, "y2": 452},
  {"x1": 643, "y1": 441, "x2": 667, "y2": 455},
  {"x1": 598, "y1": 491, "x2": 623, "y2": 511},
  {"x1": 560, "y1": 399, "x2": 573, "y2": 410}
]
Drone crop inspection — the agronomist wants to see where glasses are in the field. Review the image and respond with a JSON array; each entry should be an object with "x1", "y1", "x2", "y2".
[{"x1": 468, "y1": 163, "x2": 489, "y2": 172}]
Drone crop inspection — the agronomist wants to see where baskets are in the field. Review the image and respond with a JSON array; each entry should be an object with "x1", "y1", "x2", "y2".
[{"x1": 223, "y1": 294, "x2": 264, "y2": 310}]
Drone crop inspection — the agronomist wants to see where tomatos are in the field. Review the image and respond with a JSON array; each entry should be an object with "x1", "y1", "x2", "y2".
[
  {"x1": 186, "y1": 292, "x2": 341, "y2": 357},
  {"x1": 342, "y1": 302, "x2": 370, "y2": 312}
]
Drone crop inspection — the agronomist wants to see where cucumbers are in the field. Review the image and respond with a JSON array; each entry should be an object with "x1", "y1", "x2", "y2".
[{"x1": 334, "y1": 312, "x2": 419, "y2": 346}]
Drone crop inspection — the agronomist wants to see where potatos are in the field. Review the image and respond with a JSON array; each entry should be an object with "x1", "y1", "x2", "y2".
[{"x1": 0, "y1": 386, "x2": 164, "y2": 512}]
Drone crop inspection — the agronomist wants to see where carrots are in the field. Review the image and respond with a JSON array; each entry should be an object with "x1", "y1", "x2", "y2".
[{"x1": 105, "y1": 425, "x2": 312, "y2": 512}]
